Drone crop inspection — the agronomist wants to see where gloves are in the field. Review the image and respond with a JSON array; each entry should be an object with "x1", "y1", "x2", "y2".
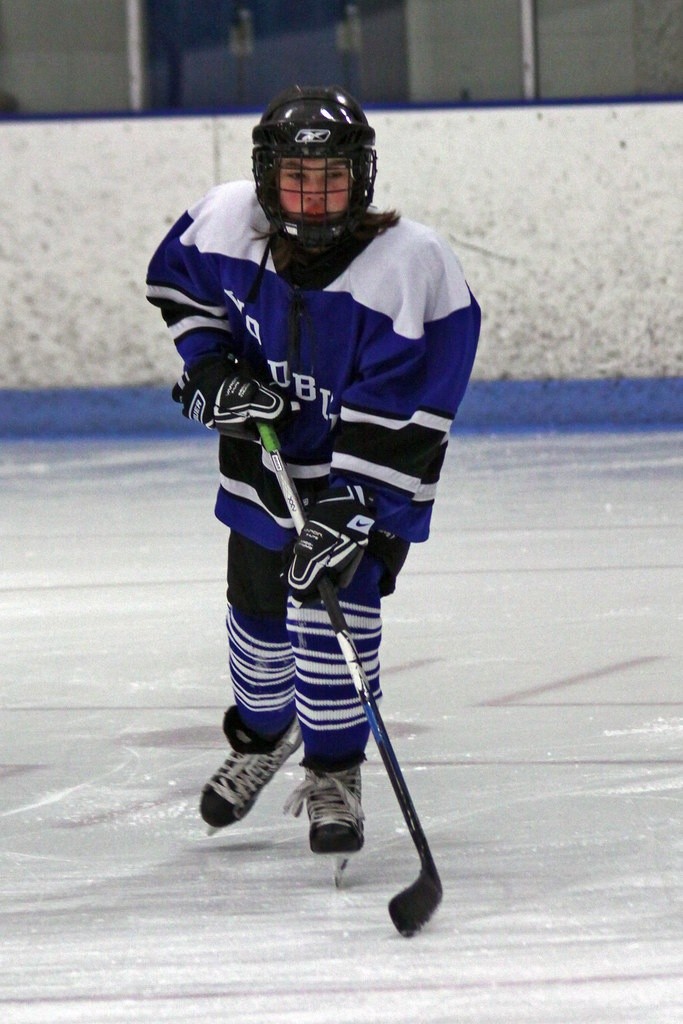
[
  {"x1": 287, "y1": 485, "x2": 374, "y2": 602},
  {"x1": 172, "y1": 335, "x2": 302, "y2": 441}
]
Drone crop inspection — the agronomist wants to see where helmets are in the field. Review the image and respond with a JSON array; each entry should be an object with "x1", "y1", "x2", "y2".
[{"x1": 251, "y1": 83, "x2": 376, "y2": 254}]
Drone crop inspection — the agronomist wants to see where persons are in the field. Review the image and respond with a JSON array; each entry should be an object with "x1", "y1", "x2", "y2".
[{"x1": 147, "y1": 84, "x2": 483, "y2": 891}]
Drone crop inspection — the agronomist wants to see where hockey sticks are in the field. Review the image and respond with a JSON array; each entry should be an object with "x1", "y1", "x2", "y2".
[{"x1": 251, "y1": 417, "x2": 452, "y2": 937}]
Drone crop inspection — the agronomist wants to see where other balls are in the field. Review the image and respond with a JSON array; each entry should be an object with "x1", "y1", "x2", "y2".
[{"x1": 333, "y1": 855, "x2": 348, "y2": 887}]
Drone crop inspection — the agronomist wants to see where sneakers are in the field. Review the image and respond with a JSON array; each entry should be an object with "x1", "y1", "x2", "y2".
[
  {"x1": 201, "y1": 719, "x2": 303, "y2": 836},
  {"x1": 284, "y1": 756, "x2": 367, "y2": 889}
]
